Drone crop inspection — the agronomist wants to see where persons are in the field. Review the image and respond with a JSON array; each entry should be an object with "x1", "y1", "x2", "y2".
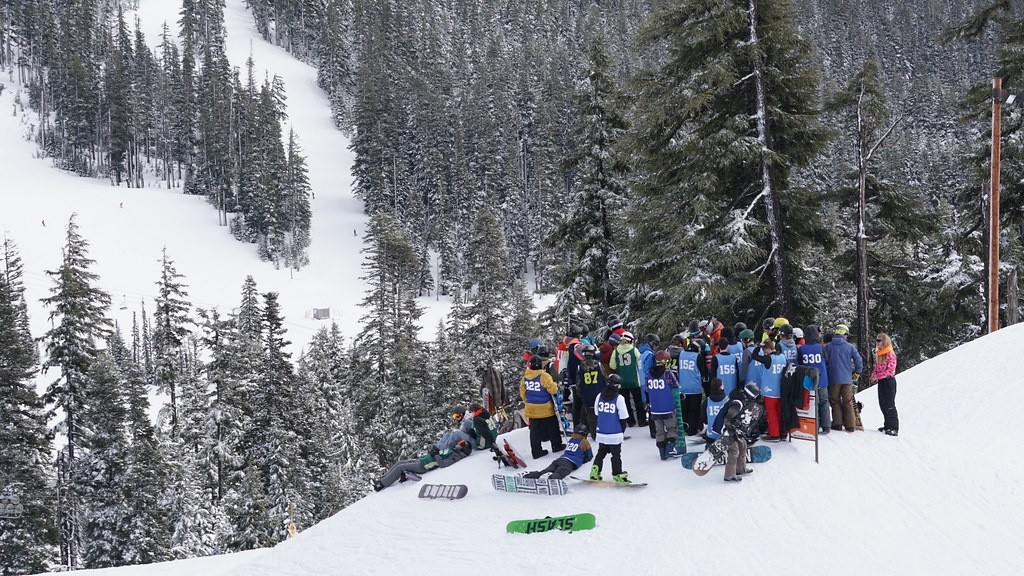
[
  {"x1": 372, "y1": 401, "x2": 498, "y2": 493},
  {"x1": 869, "y1": 333, "x2": 899, "y2": 436},
  {"x1": 518, "y1": 316, "x2": 863, "y2": 484}
]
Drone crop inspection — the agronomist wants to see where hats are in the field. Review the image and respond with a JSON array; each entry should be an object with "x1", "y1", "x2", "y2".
[
  {"x1": 569, "y1": 325, "x2": 582, "y2": 335},
  {"x1": 468, "y1": 404, "x2": 481, "y2": 413},
  {"x1": 460, "y1": 441, "x2": 473, "y2": 456},
  {"x1": 528, "y1": 339, "x2": 550, "y2": 357}
]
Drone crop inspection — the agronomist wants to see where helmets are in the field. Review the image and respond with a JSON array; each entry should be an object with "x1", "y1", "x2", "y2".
[
  {"x1": 453, "y1": 407, "x2": 465, "y2": 418},
  {"x1": 607, "y1": 374, "x2": 622, "y2": 388},
  {"x1": 607, "y1": 312, "x2": 754, "y2": 364},
  {"x1": 529, "y1": 355, "x2": 542, "y2": 368},
  {"x1": 582, "y1": 345, "x2": 597, "y2": 358},
  {"x1": 744, "y1": 384, "x2": 760, "y2": 400},
  {"x1": 763, "y1": 317, "x2": 849, "y2": 341},
  {"x1": 574, "y1": 423, "x2": 589, "y2": 435}
]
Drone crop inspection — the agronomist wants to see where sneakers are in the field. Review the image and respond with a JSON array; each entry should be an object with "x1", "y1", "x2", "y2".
[{"x1": 590, "y1": 465, "x2": 632, "y2": 482}]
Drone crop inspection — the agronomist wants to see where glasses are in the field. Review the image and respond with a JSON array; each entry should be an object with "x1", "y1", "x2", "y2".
[{"x1": 876, "y1": 339, "x2": 881, "y2": 343}]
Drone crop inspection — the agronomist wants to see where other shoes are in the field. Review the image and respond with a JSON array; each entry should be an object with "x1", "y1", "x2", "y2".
[
  {"x1": 831, "y1": 425, "x2": 842, "y2": 430},
  {"x1": 533, "y1": 444, "x2": 566, "y2": 459},
  {"x1": 780, "y1": 435, "x2": 786, "y2": 441},
  {"x1": 822, "y1": 427, "x2": 830, "y2": 434},
  {"x1": 845, "y1": 426, "x2": 854, "y2": 432},
  {"x1": 724, "y1": 476, "x2": 742, "y2": 482},
  {"x1": 370, "y1": 477, "x2": 385, "y2": 493},
  {"x1": 762, "y1": 436, "x2": 780, "y2": 442},
  {"x1": 736, "y1": 469, "x2": 753, "y2": 475},
  {"x1": 878, "y1": 426, "x2": 898, "y2": 436},
  {"x1": 659, "y1": 443, "x2": 677, "y2": 460}
]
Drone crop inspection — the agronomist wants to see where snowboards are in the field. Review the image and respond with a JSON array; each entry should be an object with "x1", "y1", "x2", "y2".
[
  {"x1": 506, "y1": 512, "x2": 596, "y2": 534},
  {"x1": 490, "y1": 438, "x2": 527, "y2": 470},
  {"x1": 550, "y1": 391, "x2": 570, "y2": 442},
  {"x1": 418, "y1": 484, "x2": 468, "y2": 499},
  {"x1": 569, "y1": 465, "x2": 649, "y2": 487},
  {"x1": 681, "y1": 445, "x2": 771, "y2": 470},
  {"x1": 491, "y1": 474, "x2": 568, "y2": 496},
  {"x1": 401, "y1": 469, "x2": 422, "y2": 482},
  {"x1": 850, "y1": 380, "x2": 865, "y2": 432},
  {"x1": 674, "y1": 386, "x2": 687, "y2": 454},
  {"x1": 692, "y1": 402, "x2": 764, "y2": 477}
]
